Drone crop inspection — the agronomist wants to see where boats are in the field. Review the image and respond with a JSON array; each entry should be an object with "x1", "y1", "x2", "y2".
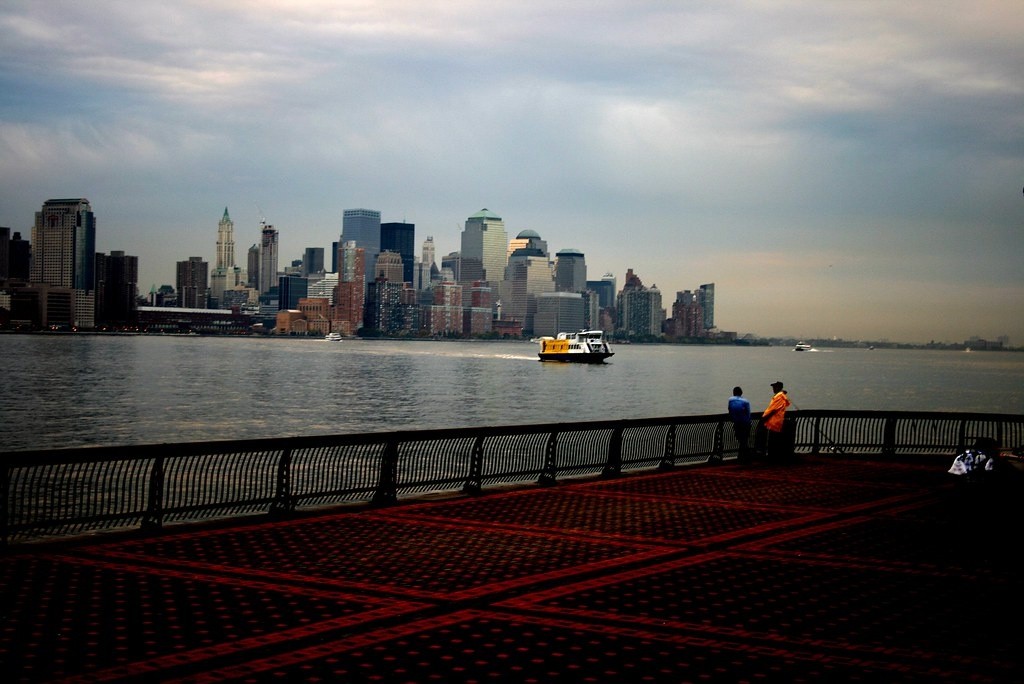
[
  {"x1": 326, "y1": 333, "x2": 342, "y2": 341},
  {"x1": 530, "y1": 336, "x2": 555, "y2": 344},
  {"x1": 537, "y1": 329, "x2": 615, "y2": 365},
  {"x1": 795, "y1": 341, "x2": 812, "y2": 351},
  {"x1": 869, "y1": 345, "x2": 875, "y2": 350}
]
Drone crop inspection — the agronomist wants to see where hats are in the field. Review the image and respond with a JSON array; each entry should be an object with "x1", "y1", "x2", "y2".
[{"x1": 770, "y1": 381, "x2": 783, "y2": 387}]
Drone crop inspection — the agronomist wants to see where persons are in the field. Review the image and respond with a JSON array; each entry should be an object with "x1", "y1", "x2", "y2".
[
  {"x1": 727, "y1": 387, "x2": 752, "y2": 465},
  {"x1": 762, "y1": 382, "x2": 791, "y2": 468}
]
